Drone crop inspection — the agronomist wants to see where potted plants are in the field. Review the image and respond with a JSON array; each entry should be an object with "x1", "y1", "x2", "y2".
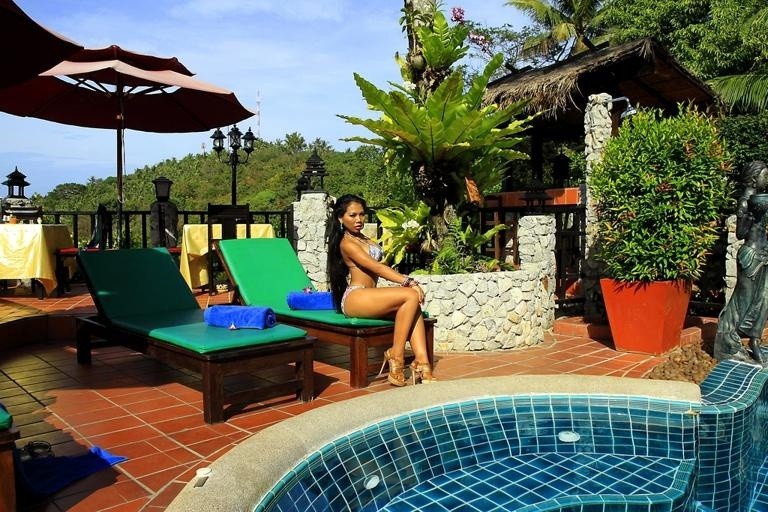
[
  {"x1": 586, "y1": 98, "x2": 739, "y2": 357},
  {"x1": 215, "y1": 272, "x2": 230, "y2": 294}
]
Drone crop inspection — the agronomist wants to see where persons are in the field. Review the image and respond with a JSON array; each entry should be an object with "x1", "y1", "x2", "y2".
[
  {"x1": 324, "y1": 193, "x2": 443, "y2": 386},
  {"x1": 712, "y1": 160, "x2": 768, "y2": 366}
]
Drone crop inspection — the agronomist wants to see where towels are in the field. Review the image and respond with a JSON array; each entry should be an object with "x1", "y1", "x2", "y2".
[
  {"x1": 286, "y1": 290, "x2": 336, "y2": 313},
  {"x1": 202, "y1": 305, "x2": 280, "y2": 329},
  {"x1": 18, "y1": 444, "x2": 129, "y2": 498}
]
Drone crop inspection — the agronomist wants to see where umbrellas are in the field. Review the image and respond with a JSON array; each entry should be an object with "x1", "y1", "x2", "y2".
[
  {"x1": 0, "y1": 42, "x2": 256, "y2": 249},
  {"x1": 0, "y1": 0, "x2": 86, "y2": 98}
]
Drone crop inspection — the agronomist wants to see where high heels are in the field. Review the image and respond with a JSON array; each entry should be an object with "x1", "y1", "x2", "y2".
[
  {"x1": 378, "y1": 348, "x2": 406, "y2": 387},
  {"x1": 409, "y1": 360, "x2": 439, "y2": 386}
]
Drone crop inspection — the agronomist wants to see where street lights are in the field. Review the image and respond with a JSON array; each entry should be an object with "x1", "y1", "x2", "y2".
[{"x1": 210, "y1": 123, "x2": 259, "y2": 206}]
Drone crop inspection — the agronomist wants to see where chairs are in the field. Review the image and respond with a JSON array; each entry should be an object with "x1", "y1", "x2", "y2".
[
  {"x1": 214, "y1": 236, "x2": 439, "y2": 388},
  {"x1": 154, "y1": 202, "x2": 185, "y2": 257},
  {"x1": 0, "y1": 400, "x2": 20, "y2": 511},
  {"x1": 54, "y1": 202, "x2": 112, "y2": 296},
  {"x1": 206, "y1": 202, "x2": 252, "y2": 295},
  {"x1": 76, "y1": 247, "x2": 319, "y2": 423}
]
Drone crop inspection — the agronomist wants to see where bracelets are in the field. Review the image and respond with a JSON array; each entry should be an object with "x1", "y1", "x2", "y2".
[{"x1": 400, "y1": 275, "x2": 420, "y2": 289}]
